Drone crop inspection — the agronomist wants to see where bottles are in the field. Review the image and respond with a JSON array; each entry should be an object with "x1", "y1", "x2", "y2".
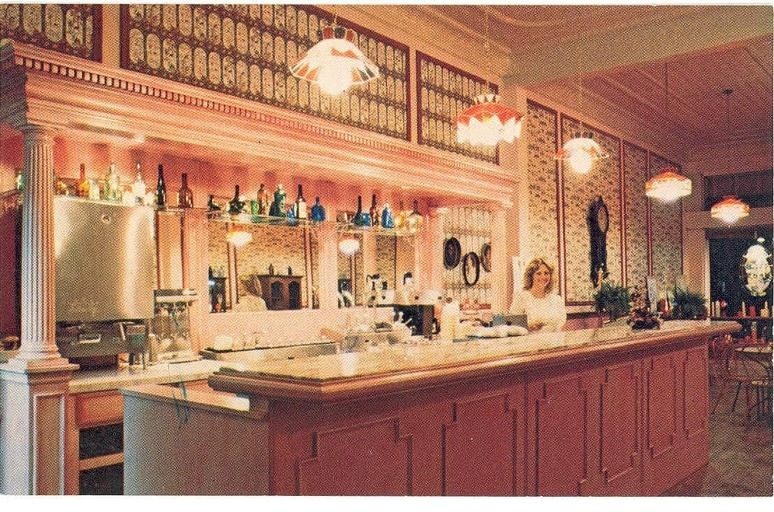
[
  {"x1": 369, "y1": 194, "x2": 381, "y2": 227},
  {"x1": 310, "y1": 196, "x2": 326, "y2": 223},
  {"x1": 295, "y1": 183, "x2": 309, "y2": 221},
  {"x1": 202, "y1": 192, "x2": 223, "y2": 219},
  {"x1": 174, "y1": 170, "x2": 194, "y2": 210},
  {"x1": 102, "y1": 162, "x2": 123, "y2": 204},
  {"x1": 348, "y1": 195, "x2": 371, "y2": 227},
  {"x1": 151, "y1": 162, "x2": 167, "y2": 208},
  {"x1": 268, "y1": 198, "x2": 277, "y2": 216},
  {"x1": 285, "y1": 203, "x2": 296, "y2": 223},
  {"x1": 382, "y1": 202, "x2": 394, "y2": 227},
  {"x1": 247, "y1": 196, "x2": 259, "y2": 220},
  {"x1": 72, "y1": 162, "x2": 92, "y2": 202},
  {"x1": 255, "y1": 183, "x2": 269, "y2": 220},
  {"x1": 129, "y1": 160, "x2": 147, "y2": 207},
  {"x1": 274, "y1": 183, "x2": 288, "y2": 216},
  {"x1": 225, "y1": 184, "x2": 245, "y2": 215},
  {"x1": 437, "y1": 295, "x2": 461, "y2": 343},
  {"x1": 396, "y1": 195, "x2": 409, "y2": 227},
  {"x1": 409, "y1": 197, "x2": 424, "y2": 232}
]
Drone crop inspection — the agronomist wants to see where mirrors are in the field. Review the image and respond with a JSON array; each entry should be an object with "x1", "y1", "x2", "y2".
[{"x1": 206, "y1": 219, "x2": 417, "y2": 314}]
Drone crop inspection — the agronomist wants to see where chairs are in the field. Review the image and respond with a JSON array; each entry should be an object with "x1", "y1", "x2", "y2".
[{"x1": 709, "y1": 332, "x2": 773, "y2": 428}]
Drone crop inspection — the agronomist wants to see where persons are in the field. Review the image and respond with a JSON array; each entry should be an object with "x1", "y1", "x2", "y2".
[
  {"x1": 233, "y1": 273, "x2": 268, "y2": 312},
  {"x1": 509, "y1": 257, "x2": 568, "y2": 332}
]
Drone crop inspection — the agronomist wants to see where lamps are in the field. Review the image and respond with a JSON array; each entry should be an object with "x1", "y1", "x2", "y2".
[
  {"x1": 645, "y1": 60, "x2": 693, "y2": 204},
  {"x1": 451, "y1": 5, "x2": 524, "y2": 148},
  {"x1": 710, "y1": 87, "x2": 751, "y2": 227},
  {"x1": 288, "y1": 5, "x2": 382, "y2": 98},
  {"x1": 552, "y1": 5, "x2": 612, "y2": 176}
]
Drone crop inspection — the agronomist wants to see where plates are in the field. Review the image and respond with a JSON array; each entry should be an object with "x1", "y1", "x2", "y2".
[{"x1": 442, "y1": 236, "x2": 492, "y2": 287}]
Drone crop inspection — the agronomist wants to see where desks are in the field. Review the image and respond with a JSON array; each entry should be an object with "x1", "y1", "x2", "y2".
[{"x1": 705, "y1": 314, "x2": 773, "y2": 338}]
